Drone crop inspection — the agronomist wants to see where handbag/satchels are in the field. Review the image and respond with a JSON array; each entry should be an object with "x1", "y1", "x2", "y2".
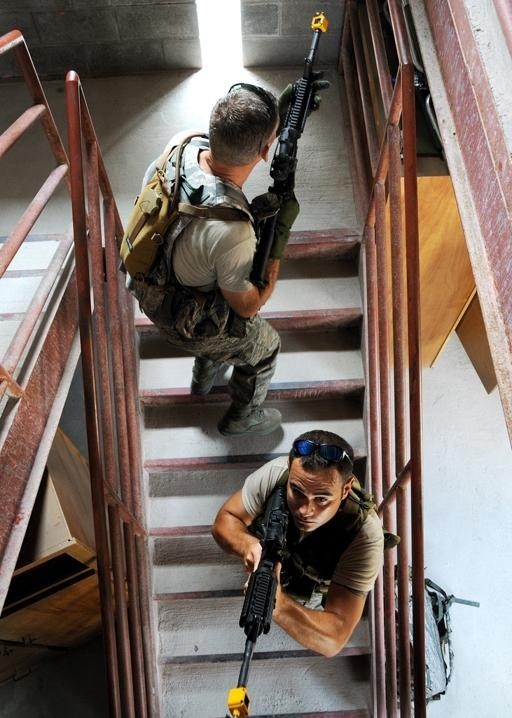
[{"x1": 174, "y1": 293, "x2": 231, "y2": 341}]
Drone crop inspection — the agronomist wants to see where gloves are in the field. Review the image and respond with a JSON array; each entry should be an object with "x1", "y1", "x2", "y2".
[
  {"x1": 276, "y1": 70, "x2": 330, "y2": 138},
  {"x1": 267, "y1": 185, "x2": 299, "y2": 260}
]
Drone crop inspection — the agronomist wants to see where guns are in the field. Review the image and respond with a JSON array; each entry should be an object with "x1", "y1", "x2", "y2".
[
  {"x1": 228, "y1": 481, "x2": 291, "y2": 718},
  {"x1": 224, "y1": 10, "x2": 327, "y2": 338}
]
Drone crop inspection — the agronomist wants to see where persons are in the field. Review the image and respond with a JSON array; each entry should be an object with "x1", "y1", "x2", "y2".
[
  {"x1": 117, "y1": 72, "x2": 331, "y2": 436},
  {"x1": 212, "y1": 430, "x2": 402, "y2": 659}
]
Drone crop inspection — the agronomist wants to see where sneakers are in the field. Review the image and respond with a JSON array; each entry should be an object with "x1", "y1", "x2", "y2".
[
  {"x1": 191, "y1": 358, "x2": 218, "y2": 395},
  {"x1": 217, "y1": 405, "x2": 282, "y2": 437}
]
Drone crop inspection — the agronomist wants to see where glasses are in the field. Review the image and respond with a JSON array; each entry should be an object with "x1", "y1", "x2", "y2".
[
  {"x1": 228, "y1": 82, "x2": 276, "y2": 123},
  {"x1": 293, "y1": 440, "x2": 353, "y2": 466}
]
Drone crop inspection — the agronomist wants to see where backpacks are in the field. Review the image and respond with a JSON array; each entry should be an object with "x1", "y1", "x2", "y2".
[
  {"x1": 119, "y1": 169, "x2": 177, "y2": 274},
  {"x1": 387, "y1": 566, "x2": 454, "y2": 703}
]
